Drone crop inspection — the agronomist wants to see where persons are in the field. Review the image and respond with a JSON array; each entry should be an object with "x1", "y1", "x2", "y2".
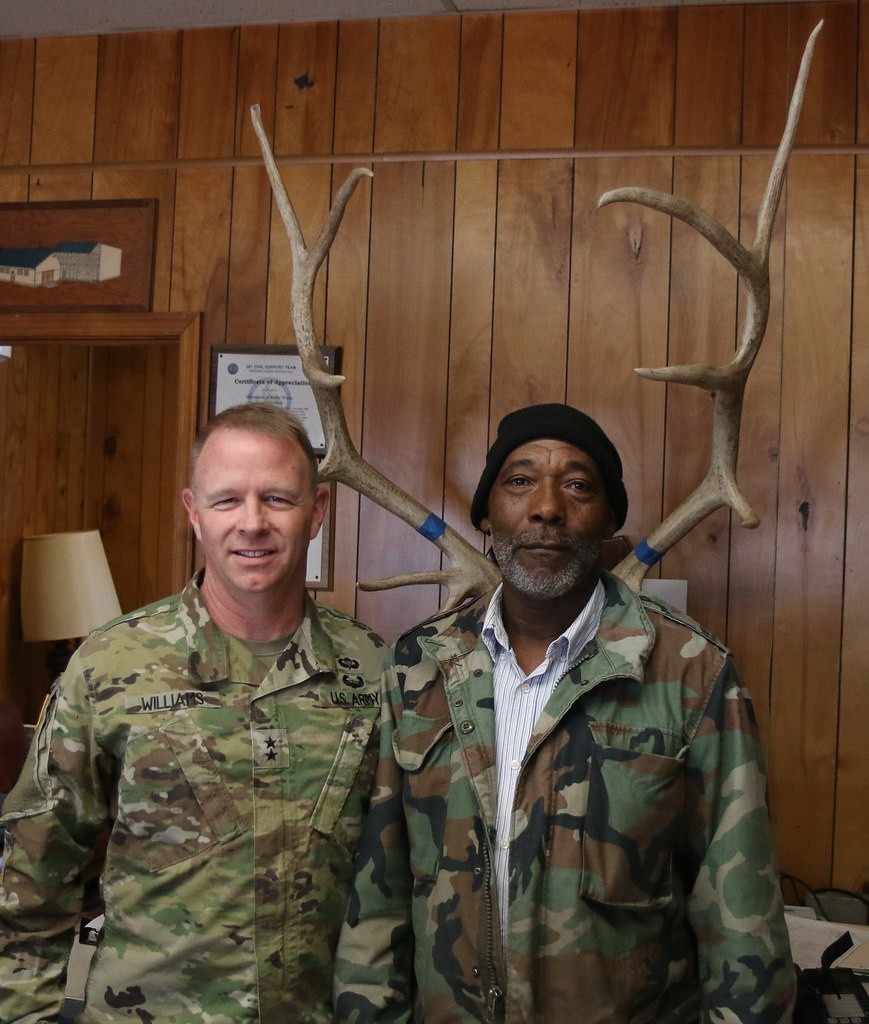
[
  {"x1": 0, "y1": 405, "x2": 426, "y2": 1024},
  {"x1": 331, "y1": 404, "x2": 802, "y2": 1024}
]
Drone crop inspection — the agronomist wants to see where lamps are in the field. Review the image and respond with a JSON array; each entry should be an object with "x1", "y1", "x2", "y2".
[{"x1": 20, "y1": 529, "x2": 125, "y2": 668}]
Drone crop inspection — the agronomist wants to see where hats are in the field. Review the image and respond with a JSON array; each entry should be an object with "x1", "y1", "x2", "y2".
[{"x1": 469, "y1": 403, "x2": 629, "y2": 535}]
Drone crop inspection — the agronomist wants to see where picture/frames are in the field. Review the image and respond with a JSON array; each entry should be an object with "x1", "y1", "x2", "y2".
[
  {"x1": 208, "y1": 342, "x2": 343, "y2": 456},
  {"x1": 298, "y1": 456, "x2": 334, "y2": 590},
  {"x1": 0, "y1": 195, "x2": 163, "y2": 312}
]
